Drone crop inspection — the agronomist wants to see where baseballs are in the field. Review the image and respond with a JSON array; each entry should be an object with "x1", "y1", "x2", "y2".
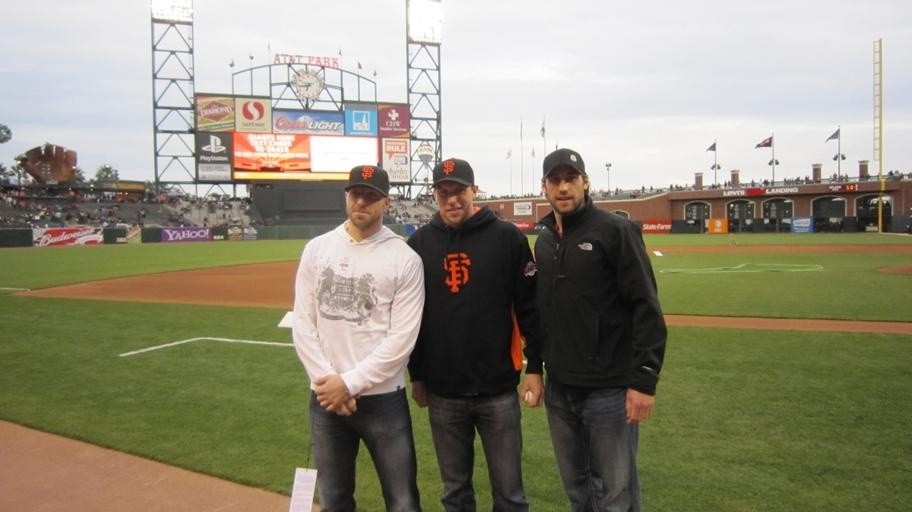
[{"x1": 524, "y1": 390, "x2": 533, "y2": 403}]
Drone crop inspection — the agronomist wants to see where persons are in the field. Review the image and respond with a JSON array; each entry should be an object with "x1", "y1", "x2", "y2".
[
  {"x1": 593, "y1": 168, "x2": 908, "y2": 199},
  {"x1": 403, "y1": 158, "x2": 547, "y2": 512},
  {"x1": 0, "y1": 193, "x2": 251, "y2": 230},
  {"x1": 388, "y1": 192, "x2": 435, "y2": 230},
  {"x1": 292, "y1": 164, "x2": 426, "y2": 512},
  {"x1": 536, "y1": 148, "x2": 668, "y2": 512}
]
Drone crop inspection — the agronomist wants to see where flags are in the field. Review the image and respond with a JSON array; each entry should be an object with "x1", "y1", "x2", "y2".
[
  {"x1": 706, "y1": 142, "x2": 716, "y2": 151},
  {"x1": 755, "y1": 136, "x2": 771, "y2": 147},
  {"x1": 825, "y1": 128, "x2": 839, "y2": 141}
]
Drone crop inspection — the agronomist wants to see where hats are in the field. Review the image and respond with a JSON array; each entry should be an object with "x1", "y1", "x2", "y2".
[
  {"x1": 543, "y1": 148, "x2": 586, "y2": 178},
  {"x1": 432, "y1": 158, "x2": 474, "y2": 187},
  {"x1": 345, "y1": 166, "x2": 390, "y2": 198}
]
